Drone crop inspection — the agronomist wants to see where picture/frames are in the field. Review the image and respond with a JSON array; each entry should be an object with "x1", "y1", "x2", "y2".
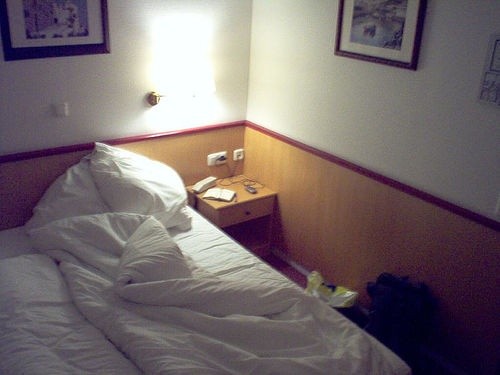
[
  {"x1": 0, "y1": 0, "x2": 111, "y2": 62},
  {"x1": 334, "y1": 0, "x2": 428, "y2": 73}
]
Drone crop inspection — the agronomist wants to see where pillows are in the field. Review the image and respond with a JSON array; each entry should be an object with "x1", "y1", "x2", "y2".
[{"x1": 29, "y1": 142, "x2": 190, "y2": 231}]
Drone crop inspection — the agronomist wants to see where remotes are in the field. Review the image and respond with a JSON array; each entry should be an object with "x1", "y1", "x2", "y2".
[{"x1": 244, "y1": 185, "x2": 257, "y2": 194}]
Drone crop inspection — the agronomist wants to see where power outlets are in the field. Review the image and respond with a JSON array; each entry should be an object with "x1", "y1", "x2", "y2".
[{"x1": 208, "y1": 151, "x2": 228, "y2": 167}]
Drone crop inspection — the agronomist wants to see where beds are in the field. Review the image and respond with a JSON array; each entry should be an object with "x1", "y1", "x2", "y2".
[{"x1": 1, "y1": 206, "x2": 412, "y2": 374}]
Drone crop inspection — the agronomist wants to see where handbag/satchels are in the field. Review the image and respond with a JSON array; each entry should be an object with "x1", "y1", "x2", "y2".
[{"x1": 304, "y1": 271, "x2": 359, "y2": 312}]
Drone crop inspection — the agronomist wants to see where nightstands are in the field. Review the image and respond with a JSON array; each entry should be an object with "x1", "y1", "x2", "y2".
[{"x1": 185, "y1": 174, "x2": 276, "y2": 260}]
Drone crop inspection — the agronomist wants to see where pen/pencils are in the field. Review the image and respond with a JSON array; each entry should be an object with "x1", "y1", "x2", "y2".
[{"x1": 234, "y1": 193, "x2": 237, "y2": 202}]
